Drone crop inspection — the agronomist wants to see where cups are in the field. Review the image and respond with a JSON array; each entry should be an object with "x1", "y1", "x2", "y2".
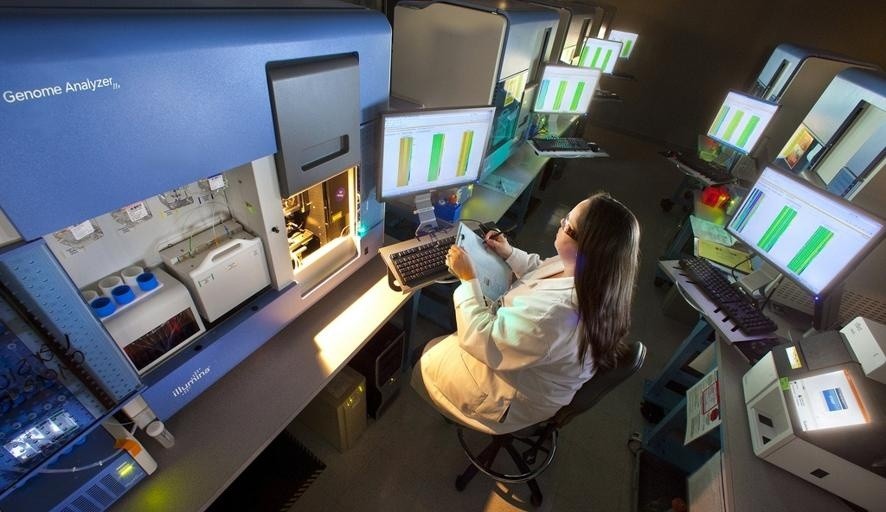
[{"x1": 79, "y1": 262, "x2": 158, "y2": 316}]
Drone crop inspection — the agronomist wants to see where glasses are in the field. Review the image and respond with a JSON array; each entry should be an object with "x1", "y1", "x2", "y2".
[{"x1": 559, "y1": 218, "x2": 579, "y2": 243}]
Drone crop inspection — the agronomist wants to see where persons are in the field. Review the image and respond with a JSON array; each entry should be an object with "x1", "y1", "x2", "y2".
[{"x1": 406, "y1": 191, "x2": 640, "y2": 435}]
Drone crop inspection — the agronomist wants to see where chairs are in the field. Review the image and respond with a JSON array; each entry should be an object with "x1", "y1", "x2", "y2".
[{"x1": 436, "y1": 337, "x2": 651, "y2": 509}]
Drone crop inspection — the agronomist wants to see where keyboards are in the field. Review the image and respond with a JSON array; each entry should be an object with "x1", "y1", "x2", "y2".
[
  {"x1": 678, "y1": 256, "x2": 778, "y2": 335},
  {"x1": 533, "y1": 137, "x2": 589, "y2": 151},
  {"x1": 389, "y1": 228, "x2": 486, "y2": 286},
  {"x1": 678, "y1": 154, "x2": 732, "y2": 181}
]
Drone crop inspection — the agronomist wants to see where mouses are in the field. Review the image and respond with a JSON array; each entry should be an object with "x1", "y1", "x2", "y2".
[
  {"x1": 612, "y1": 93, "x2": 618, "y2": 98},
  {"x1": 589, "y1": 142, "x2": 599, "y2": 152}
]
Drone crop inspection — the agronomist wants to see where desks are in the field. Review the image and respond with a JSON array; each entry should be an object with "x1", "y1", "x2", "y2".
[
  {"x1": 92, "y1": 229, "x2": 424, "y2": 511},
  {"x1": 412, "y1": 106, "x2": 583, "y2": 340},
  {"x1": 629, "y1": 199, "x2": 865, "y2": 512}
]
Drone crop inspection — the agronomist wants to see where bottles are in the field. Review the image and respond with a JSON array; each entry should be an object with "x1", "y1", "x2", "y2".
[{"x1": 144, "y1": 420, "x2": 175, "y2": 448}]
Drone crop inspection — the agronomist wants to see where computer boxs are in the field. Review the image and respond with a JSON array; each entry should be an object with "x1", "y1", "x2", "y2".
[
  {"x1": 311, "y1": 364, "x2": 368, "y2": 452},
  {"x1": 348, "y1": 321, "x2": 407, "y2": 422}
]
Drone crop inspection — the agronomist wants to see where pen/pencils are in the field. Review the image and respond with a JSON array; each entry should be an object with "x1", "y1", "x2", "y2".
[
  {"x1": 482, "y1": 224, "x2": 517, "y2": 244},
  {"x1": 500, "y1": 177, "x2": 507, "y2": 193}
]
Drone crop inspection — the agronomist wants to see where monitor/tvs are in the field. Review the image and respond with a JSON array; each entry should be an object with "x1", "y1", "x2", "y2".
[
  {"x1": 577, "y1": 36, "x2": 623, "y2": 75},
  {"x1": 375, "y1": 105, "x2": 496, "y2": 202},
  {"x1": 532, "y1": 63, "x2": 601, "y2": 116},
  {"x1": 706, "y1": 88, "x2": 782, "y2": 156},
  {"x1": 787, "y1": 367, "x2": 873, "y2": 433},
  {"x1": 609, "y1": 29, "x2": 639, "y2": 59},
  {"x1": 723, "y1": 163, "x2": 886, "y2": 300}
]
